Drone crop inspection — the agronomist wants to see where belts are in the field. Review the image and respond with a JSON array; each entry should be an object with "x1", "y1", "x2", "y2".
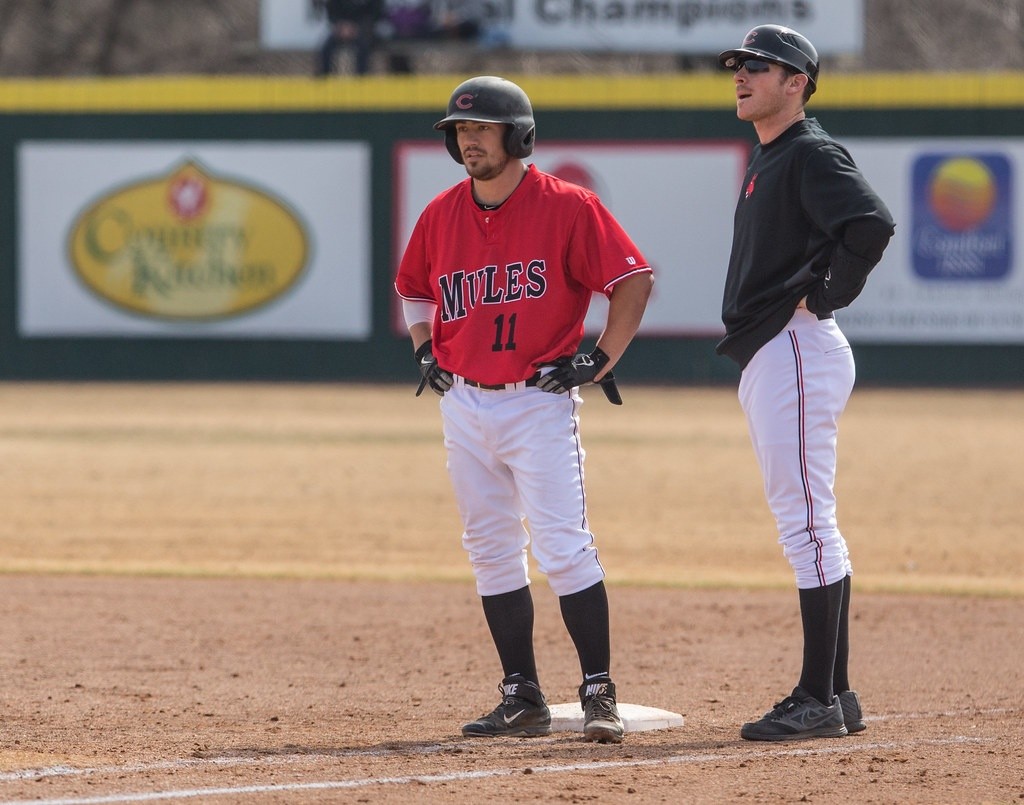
[
  {"x1": 817, "y1": 309, "x2": 836, "y2": 322},
  {"x1": 465, "y1": 372, "x2": 543, "y2": 391}
]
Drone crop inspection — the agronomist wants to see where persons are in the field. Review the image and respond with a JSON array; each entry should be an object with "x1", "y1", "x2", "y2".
[
  {"x1": 716, "y1": 23, "x2": 895, "y2": 741},
  {"x1": 397, "y1": 75, "x2": 655, "y2": 740},
  {"x1": 313, "y1": 0, "x2": 511, "y2": 77}
]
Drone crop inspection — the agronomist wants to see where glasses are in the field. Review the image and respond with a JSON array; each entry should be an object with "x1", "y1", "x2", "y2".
[{"x1": 726, "y1": 57, "x2": 797, "y2": 75}]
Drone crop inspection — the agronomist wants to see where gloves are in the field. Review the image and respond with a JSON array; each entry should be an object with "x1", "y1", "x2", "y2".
[
  {"x1": 537, "y1": 343, "x2": 623, "y2": 406},
  {"x1": 413, "y1": 339, "x2": 454, "y2": 397}
]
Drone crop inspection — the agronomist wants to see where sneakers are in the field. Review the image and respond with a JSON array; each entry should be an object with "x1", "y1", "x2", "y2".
[
  {"x1": 579, "y1": 674, "x2": 624, "y2": 742},
  {"x1": 741, "y1": 685, "x2": 848, "y2": 742},
  {"x1": 839, "y1": 690, "x2": 866, "y2": 732},
  {"x1": 461, "y1": 673, "x2": 553, "y2": 737}
]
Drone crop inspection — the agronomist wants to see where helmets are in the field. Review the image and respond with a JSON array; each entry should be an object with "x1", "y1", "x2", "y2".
[
  {"x1": 717, "y1": 24, "x2": 820, "y2": 94},
  {"x1": 432, "y1": 75, "x2": 535, "y2": 165}
]
